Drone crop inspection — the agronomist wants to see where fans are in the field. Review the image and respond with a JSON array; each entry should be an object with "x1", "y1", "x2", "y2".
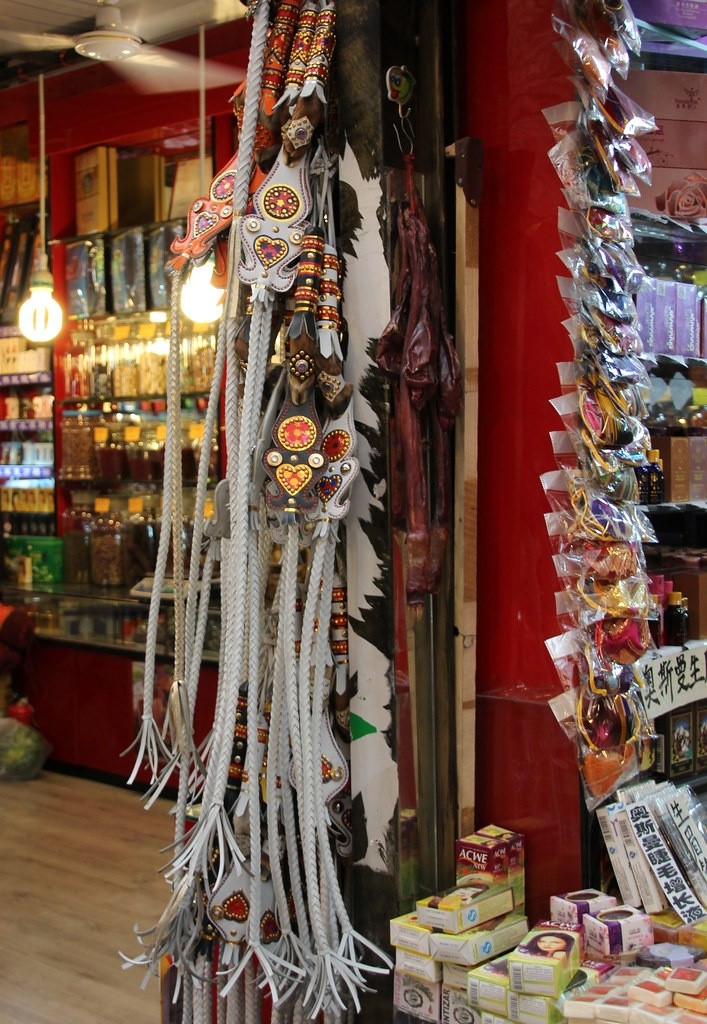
[{"x1": 1, "y1": 1, "x2": 250, "y2": 96}]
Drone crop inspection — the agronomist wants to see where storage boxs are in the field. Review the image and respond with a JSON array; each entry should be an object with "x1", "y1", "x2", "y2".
[
  {"x1": 635, "y1": 274, "x2": 701, "y2": 358},
  {"x1": 389, "y1": 699, "x2": 707, "y2": 1024},
  {"x1": 650, "y1": 436, "x2": 706, "y2": 504},
  {"x1": 606, "y1": 0, "x2": 706, "y2": 220},
  {"x1": 1, "y1": 337, "x2": 50, "y2": 373},
  {"x1": 0, "y1": 124, "x2": 48, "y2": 206},
  {"x1": 673, "y1": 572, "x2": 707, "y2": 640},
  {"x1": 64, "y1": 146, "x2": 213, "y2": 318}
]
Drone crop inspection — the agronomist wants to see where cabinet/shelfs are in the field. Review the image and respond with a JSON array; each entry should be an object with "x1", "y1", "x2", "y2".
[
  {"x1": 463, "y1": 0, "x2": 706, "y2": 925},
  {"x1": 0, "y1": 48, "x2": 224, "y2": 795}
]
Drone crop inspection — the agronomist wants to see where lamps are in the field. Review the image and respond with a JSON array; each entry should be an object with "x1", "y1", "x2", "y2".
[
  {"x1": 15, "y1": 73, "x2": 64, "y2": 344},
  {"x1": 179, "y1": 26, "x2": 226, "y2": 324}
]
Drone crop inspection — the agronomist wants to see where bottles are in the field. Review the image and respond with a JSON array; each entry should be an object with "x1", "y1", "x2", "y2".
[
  {"x1": 54, "y1": 326, "x2": 223, "y2": 590},
  {"x1": 647, "y1": 575, "x2": 688, "y2": 648},
  {"x1": 637, "y1": 403, "x2": 707, "y2": 506}
]
